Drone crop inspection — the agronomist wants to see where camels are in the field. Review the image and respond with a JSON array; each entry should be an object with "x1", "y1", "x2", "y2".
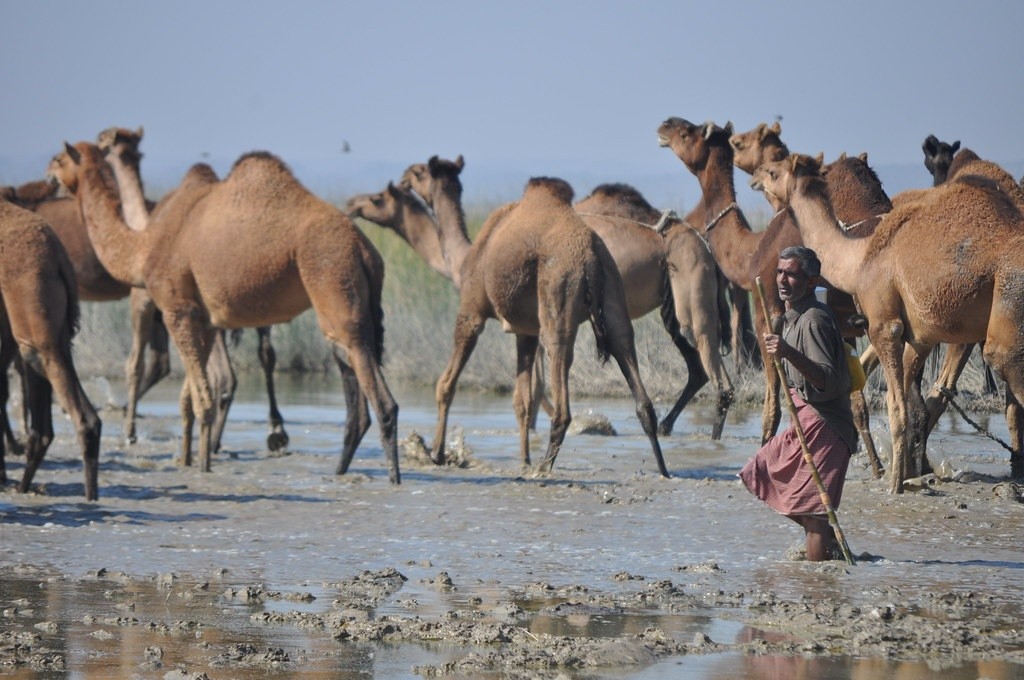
[{"x1": 0, "y1": 116, "x2": 1024, "y2": 505}]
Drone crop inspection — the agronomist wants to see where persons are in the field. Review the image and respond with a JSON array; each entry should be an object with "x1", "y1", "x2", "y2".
[{"x1": 738, "y1": 246, "x2": 859, "y2": 561}]
